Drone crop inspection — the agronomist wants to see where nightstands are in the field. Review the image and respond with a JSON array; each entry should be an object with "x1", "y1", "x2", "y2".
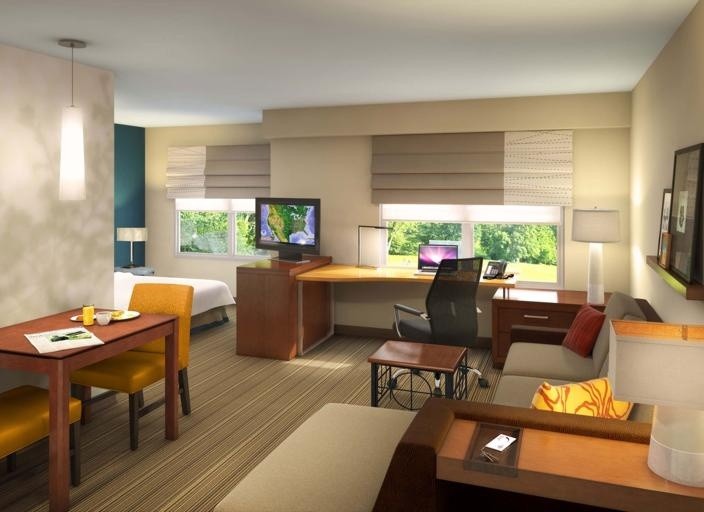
[{"x1": 114, "y1": 264, "x2": 156, "y2": 276}]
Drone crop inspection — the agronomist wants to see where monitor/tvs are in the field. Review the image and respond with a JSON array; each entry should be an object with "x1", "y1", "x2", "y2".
[
  {"x1": 418, "y1": 244, "x2": 458, "y2": 272},
  {"x1": 255, "y1": 197, "x2": 320, "y2": 264}
]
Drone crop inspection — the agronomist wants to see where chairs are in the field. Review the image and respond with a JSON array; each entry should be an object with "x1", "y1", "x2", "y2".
[
  {"x1": 387, "y1": 256, "x2": 488, "y2": 397},
  {"x1": 69, "y1": 283, "x2": 195, "y2": 451},
  {"x1": 0, "y1": 384, "x2": 81, "y2": 486}
]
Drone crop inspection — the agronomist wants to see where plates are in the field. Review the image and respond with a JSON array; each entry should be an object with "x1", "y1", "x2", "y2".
[
  {"x1": 109, "y1": 310, "x2": 140, "y2": 321},
  {"x1": 69, "y1": 314, "x2": 98, "y2": 322}
]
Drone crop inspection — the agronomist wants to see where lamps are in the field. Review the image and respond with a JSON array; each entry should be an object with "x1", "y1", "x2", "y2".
[
  {"x1": 56, "y1": 39, "x2": 86, "y2": 200},
  {"x1": 356, "y1": 225, "x2": 393, "y2": 269},
  {"x1": 116, "y1": 227, "x2": 148, "y2": 268},
  {"x1": 610, "y1": 318, "x2": 703, "y2": 490},
  {"x1": 571, "y1": 207, "x2": 622, "y2": 305}
]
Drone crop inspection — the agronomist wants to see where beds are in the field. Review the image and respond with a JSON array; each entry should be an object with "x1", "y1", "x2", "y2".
[{"x1": 113, "y1": 272, "x2": 227, "y2": 335}]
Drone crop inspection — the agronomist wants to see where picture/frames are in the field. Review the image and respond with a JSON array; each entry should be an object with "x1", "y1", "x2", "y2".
[
  {"x1": 668, "y1": 142, "x2": 704, "y2": 284},
  {"x1": 657, "y1": 188, "x2": 672, "y2": 260},
  {"x1": 659, "y1": 232, "x2": 673, "y2": 269}
]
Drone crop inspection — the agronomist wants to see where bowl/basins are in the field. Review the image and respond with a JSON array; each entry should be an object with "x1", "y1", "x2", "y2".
[{"x1": 97, "y1": 312, "x2": 111, "y2": 325}]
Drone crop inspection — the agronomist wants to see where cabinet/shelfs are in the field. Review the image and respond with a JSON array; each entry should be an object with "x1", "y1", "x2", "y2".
[
  {"x1": 492, "y1": 287, "x2": 613, "y2": 369},
  {"x1": 646, "y1": 256, "x2": 703, "y2": 300},
  {"x1": 371, "y1": 398, "x2": 704, "y2": 512},
  {"x1": 236, "y1": 256, "x2": 332, "y2": 361}
]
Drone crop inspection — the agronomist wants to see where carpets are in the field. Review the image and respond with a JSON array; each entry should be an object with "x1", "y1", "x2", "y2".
[{"x1": 214, "y1": 403, "x2": 418, "y2": 512}]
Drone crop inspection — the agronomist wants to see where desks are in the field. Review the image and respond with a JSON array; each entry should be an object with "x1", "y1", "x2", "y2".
[
  {"x1": 295, "y1": 264, "x2": 517, "y2": 357},
  {"x1": 368, "y1": 340, "x2": 469, "y2": 412}
]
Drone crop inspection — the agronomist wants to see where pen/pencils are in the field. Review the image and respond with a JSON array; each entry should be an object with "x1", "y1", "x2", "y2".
[{"x1": 51, "y1": 331, "x2": 90, "y2": 342}]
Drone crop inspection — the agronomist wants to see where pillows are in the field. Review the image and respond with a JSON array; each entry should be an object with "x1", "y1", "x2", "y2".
[
  {"x1": 529, "y1": 378, "x2": 633, "y2": 420},
  {"x1": 562, "y1": 303, "x2": 606, "y2": 358}
]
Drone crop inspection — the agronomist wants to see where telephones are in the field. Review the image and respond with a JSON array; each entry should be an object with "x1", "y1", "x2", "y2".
[{"x1": 483, "y1": 261, "x2": 513, "y2": 279}]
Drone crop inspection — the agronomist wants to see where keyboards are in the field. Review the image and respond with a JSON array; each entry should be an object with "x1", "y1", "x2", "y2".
[{"x1": 415, "y1": 269, "x2": 457, "y2": 275}]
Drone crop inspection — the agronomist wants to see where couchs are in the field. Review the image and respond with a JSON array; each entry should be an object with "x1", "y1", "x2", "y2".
[{"x1": 491, "y1": 290, "x2": 665, "y2": 424}]
